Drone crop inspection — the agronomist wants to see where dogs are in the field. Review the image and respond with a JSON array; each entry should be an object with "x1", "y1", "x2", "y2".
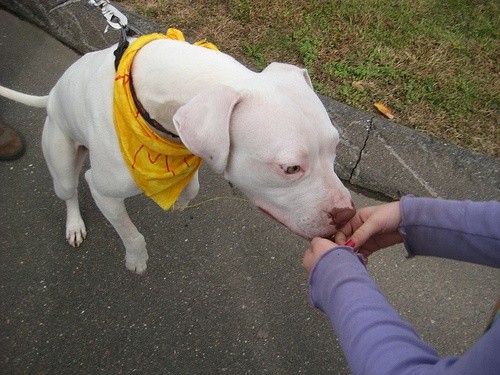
[{"x1": 0, "y1": 35, "x2": 355, "y2": 275}]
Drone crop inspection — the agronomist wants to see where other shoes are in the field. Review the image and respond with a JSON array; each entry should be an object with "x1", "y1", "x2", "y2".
[{"x1": 0, "y1": 122, "x2": 26, "y2": 161}]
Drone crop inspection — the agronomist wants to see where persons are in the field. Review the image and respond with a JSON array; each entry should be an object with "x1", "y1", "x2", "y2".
[{"x1": 302, "y1": 195, "x2": 500, "y2": 375}]
